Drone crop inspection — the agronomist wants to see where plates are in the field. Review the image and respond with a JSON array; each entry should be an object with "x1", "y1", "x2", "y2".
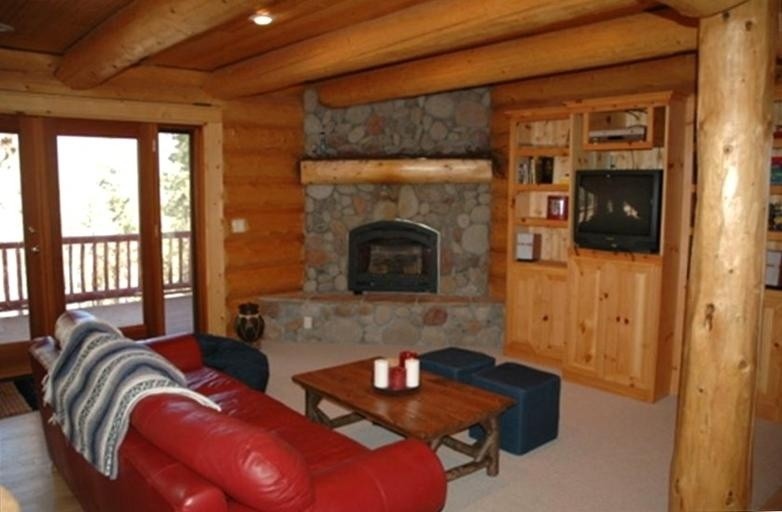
[{"x1": 375, "y1": 387, "x2": 422, "y2": 397}]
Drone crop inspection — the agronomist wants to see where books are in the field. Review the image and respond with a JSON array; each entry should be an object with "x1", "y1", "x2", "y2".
[{"x1": 519, "y1": 158, "x2": 545, "y2": 185}]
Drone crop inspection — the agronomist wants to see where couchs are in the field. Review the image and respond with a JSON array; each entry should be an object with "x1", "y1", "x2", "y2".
[{"x1": 26, "y1": 309, "x2": 448, "y2": 512}]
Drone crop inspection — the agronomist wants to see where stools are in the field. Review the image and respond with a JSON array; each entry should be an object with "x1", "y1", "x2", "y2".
[{"x1": 417, "y1": 345, "x2": 563, "y2": 455}]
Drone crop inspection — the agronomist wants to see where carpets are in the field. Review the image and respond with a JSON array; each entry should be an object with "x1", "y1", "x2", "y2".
[{"x1": 0, "y1": 373, "x2": 41, "y2": 422}]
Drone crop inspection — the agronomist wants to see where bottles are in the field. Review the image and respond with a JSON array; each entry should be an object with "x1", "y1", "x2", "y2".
[{"x1": 373, "y1": 352, "x2": 421, "y2": 390}]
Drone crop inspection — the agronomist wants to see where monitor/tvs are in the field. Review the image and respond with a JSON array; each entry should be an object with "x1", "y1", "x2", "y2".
[{"x1": 574, "y1": 169, "x2": 663, "y2": 254}]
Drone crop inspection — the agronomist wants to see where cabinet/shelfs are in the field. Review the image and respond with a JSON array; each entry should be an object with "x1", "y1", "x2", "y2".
[
  {"x1": 568, "y1": 92, "x2": 686, "y2": 404},
  {"x1": 502, "y1": 92, "x2": 577, "y2": 376}
]
